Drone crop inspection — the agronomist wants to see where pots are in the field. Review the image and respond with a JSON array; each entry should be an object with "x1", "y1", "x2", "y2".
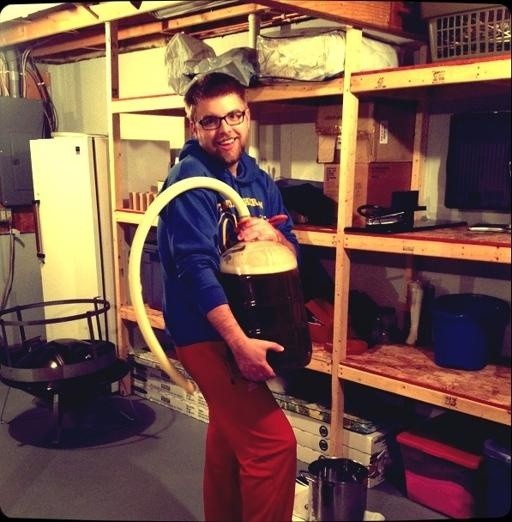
[{"x1": 297, "y1": 454, "x2": 381, "y2": 522}]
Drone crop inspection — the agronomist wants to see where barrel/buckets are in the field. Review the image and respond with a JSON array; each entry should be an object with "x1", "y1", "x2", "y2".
[
  {"x1": 432, "y1": 292, "x2": 497, "y2": 371},
  {"x1": 299, "y1": 458, "x2": 368, "y2": 522}
]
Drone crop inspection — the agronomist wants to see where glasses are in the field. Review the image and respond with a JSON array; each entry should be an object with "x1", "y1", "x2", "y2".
[{"x1": 199, "y1": 112, "x2": 245, "y2": 131}]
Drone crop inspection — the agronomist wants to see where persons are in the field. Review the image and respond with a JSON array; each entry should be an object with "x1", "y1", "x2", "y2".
[{"x1": 156, "y1": 71, "x2": 300, "y2": 522}]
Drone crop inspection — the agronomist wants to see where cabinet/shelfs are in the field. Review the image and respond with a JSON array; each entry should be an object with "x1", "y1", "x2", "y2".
[{"x1": 104, "y1": 3, "x2": 512, "y2": 522}]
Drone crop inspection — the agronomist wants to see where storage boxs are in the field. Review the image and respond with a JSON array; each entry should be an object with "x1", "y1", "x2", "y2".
[
  {"x1": 316, "y1": 100, "x2": 414, "y2": 162},
  {"x1": 323, "y1": 163, "x2": 413, "y2": 228},
  {"x1": 483, "y1": 424, "x2": 512, "y2": 522},
  {"x1": 419, "y1": 2, "x2": 511, "y2": 19},
  {"x1": 398, "y1": 412, "x2": 483, "y2": 522}
]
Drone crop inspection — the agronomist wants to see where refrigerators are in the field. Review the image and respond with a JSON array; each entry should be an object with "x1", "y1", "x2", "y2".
[{"x1": 29, "y1": 131, "x2": 172, "y2": 394}]
